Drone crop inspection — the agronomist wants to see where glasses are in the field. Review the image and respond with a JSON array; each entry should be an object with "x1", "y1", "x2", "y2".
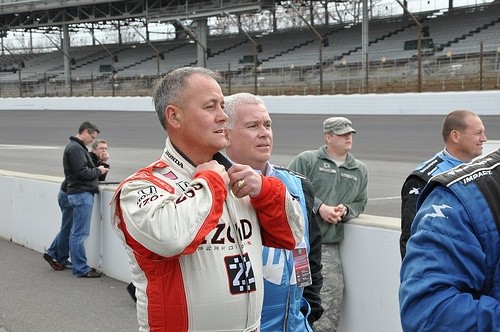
[{"x1": 86, "y1": 129, "x2": 95, "y2": 140}]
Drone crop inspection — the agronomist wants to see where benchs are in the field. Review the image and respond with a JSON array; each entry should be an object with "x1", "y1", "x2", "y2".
[{"x1": 0, "y1": 0, "x2": 500, "y2": 85}]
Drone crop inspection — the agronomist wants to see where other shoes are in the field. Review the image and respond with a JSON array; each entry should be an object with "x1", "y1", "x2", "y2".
[{"x1": 43, "y1": 253, "x2": 62, "y2": 270}]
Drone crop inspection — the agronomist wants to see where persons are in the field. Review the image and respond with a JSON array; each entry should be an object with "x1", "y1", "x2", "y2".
[
  {"x1": 219, "y1": 91, "x2": 324, "y2": 332},
  {"x1": 42, "y1": 139, "x2": 110, "y2": 271},
  {"x1": 108, "y1": 66, "x2": 307, "y2": 331},
  {"x1": 285, "y1": 116, "x2": 368, "y2": 332},
  {"x1": 62, "y1": 121, "x2": 108, "y2": 278},
  {"x1": 399, "y1": 110, "x2": 488, "y2": 262},
  {"x1": 399, "y1": 144, "x2": 500, "y2": 332}
]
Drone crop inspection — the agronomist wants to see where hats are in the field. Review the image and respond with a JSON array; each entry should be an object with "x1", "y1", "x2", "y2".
[{"x1": 323, "y1": 116, "x2": 357, "y2": 136}]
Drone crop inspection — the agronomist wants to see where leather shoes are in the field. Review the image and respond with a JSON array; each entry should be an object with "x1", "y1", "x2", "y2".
[{"x1": 78, "y1": 270, "x2": 101, "y2": 277}]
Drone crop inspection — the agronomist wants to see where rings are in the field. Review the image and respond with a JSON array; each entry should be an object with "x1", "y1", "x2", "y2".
[{"x1": 237, "y1": 178, "x2": 247, "y2": 189}]
